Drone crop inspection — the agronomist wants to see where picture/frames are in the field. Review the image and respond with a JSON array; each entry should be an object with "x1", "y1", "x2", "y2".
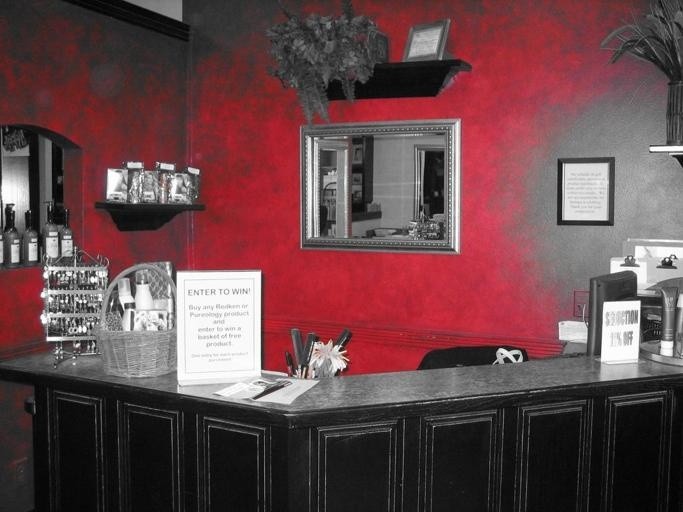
[
  {"x1": 406, "y1": 17, "x2": 452, "y2": 60},
  {"x1": 555, "y1": 157, "x2": 615, "y2": 226}
]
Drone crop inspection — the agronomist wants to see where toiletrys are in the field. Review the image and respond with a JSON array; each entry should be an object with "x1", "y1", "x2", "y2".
[{"x1": 660, "y1": 286, "x2": 678, "y2": 349}]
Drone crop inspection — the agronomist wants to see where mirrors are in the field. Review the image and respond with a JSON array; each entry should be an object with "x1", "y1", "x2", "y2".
[{"x1": 299, "y1": 118, "x2": 459, "y2": 253}]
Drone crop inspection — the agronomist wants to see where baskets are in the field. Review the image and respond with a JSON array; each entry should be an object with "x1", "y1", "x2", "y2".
[{"x1": 88, "y1": 261, "x2": 179, "y2": 380}]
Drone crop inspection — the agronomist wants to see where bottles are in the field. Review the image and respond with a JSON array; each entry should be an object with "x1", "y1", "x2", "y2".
[
  {"x1": 118, "y1": 271, "x2": 155, "y2": 330},
  {"x1": 4, "y1": 200, "x2": 75, "y2": 268}
]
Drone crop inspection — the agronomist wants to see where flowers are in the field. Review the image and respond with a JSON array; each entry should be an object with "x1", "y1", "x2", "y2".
[{"x1": 268, "y1": 1, "x2": 386, "y2": 125}]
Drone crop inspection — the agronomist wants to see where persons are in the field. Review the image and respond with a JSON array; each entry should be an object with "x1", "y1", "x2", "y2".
[{"x1": 107, "y1": 169, "x2": 192, "y2": 201}]
